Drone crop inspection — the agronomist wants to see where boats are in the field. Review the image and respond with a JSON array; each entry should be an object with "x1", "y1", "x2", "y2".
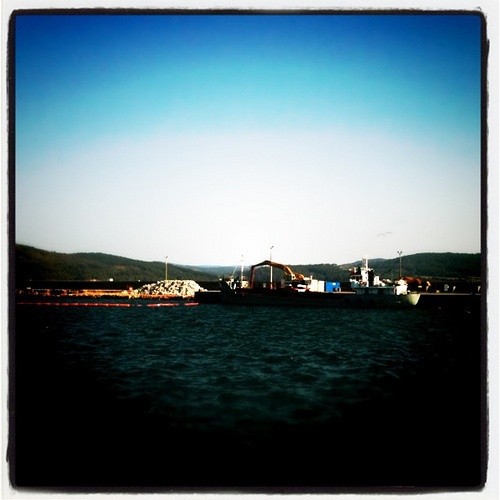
[{"x1": 194, "y1": 258, "x2": 422, "y2": 312}]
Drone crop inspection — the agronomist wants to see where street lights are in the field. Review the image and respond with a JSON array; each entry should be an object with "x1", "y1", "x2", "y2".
[
  {"x1": 270, "y1": 245, "x2": 275, "y2": 289},
  {"x1": 165, "y1": 255, "x2": 168, "y2": 280},
  {"x1": 398, "y1": 251, "x2": 404, "y2": 277}
]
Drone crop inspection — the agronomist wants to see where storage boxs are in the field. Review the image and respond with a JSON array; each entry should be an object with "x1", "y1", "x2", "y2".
[{"x1": 305, "y1": 277, "x2": 372, "y2": 294}]
